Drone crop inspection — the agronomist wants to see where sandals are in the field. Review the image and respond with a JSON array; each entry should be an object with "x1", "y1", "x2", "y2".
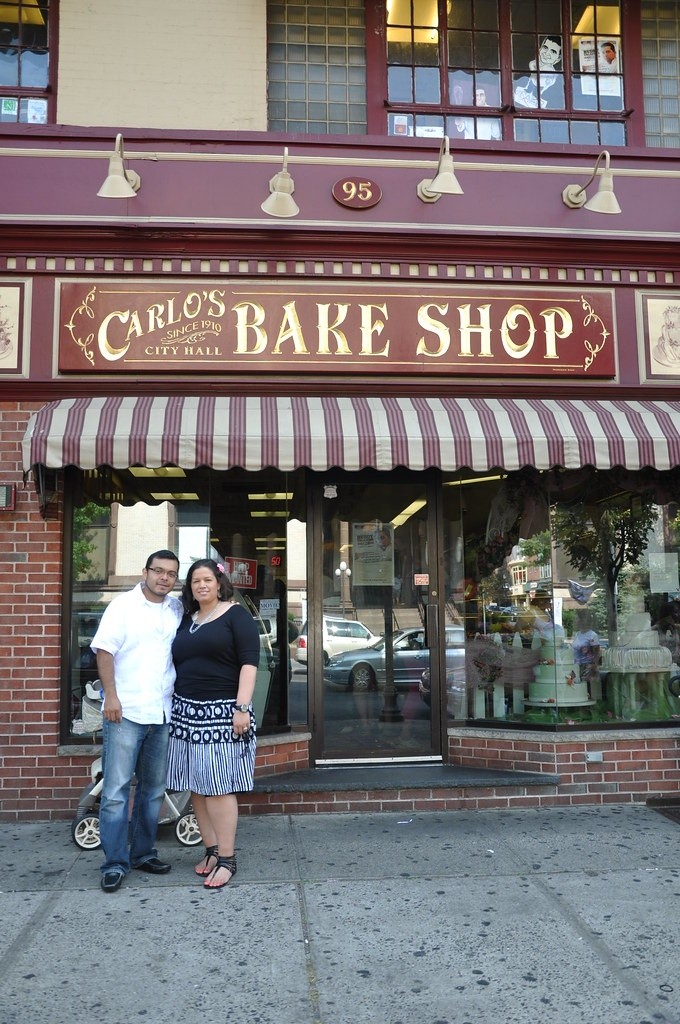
[
  {"x1": 204, "y1": 854, "x2": 237, "y2": 889},
  {"x1": 196, "y1": 844, "x2": 218, "y2": 878}
]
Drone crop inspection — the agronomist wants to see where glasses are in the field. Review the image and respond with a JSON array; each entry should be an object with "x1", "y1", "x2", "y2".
[{"x1": 148, "y1": 567, "x2": 178, "y2": 579}]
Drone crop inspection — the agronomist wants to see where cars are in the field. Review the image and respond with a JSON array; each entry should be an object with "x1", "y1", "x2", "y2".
[
  {"x1": 486, "y1": 605, "x2": 528, "y2": 621},
  {"x1": 321, "y1": 623, "x2": 465, "y2": 693},
  {"x1": 418, "y1": 664, "x2": 468, "y2": 715},
  {"x1": 72, "y1": 611, "x2": 107, "y2": 666}
]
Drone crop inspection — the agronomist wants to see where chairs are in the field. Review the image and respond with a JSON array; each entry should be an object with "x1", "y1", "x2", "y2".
[{"x1": 408, "y1": 639, "x2": 421, "y2": 650}]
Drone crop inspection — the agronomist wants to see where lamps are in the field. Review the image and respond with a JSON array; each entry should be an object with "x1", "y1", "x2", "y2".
[
  {"x1": 416, "y1": 136, "x2": 464, "y2": 203},
  {"x1": 96, "y1": 134, "x2": 141, "y2": 198},
  {"x1": 562, "y1": 150, "x2": 622, "y2": 215},
  {"x1": 260, "y1": 146, "x2": 301, "y2": 217}
]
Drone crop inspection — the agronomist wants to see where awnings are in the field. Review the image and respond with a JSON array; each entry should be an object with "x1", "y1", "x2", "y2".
[{"x1": 19, "y1": 397, "x2": 680, "y2": 493}]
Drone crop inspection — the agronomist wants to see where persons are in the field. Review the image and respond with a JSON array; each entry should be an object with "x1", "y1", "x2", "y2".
[
  {"x1": 499, "y1": 589, "x2": 680, "y2": 680},
  {"x1": 375, "y1": 528, "x2": 393, "y2": 560},
  {"x1": 166, "y1": 558, "x2": 261, "y2": 889},
  {"x1": 402, "y1": 635, "x2": 421, "y2": 650},
  {"x1": 582, "y1": 42, "x2": 618, "y2": 74},
  {"x1": 391, "y1": 572, "x2": 404, "y2": 605},
  {"x1": 89, "y1": 550, "x2": 184, "y2": 893}
]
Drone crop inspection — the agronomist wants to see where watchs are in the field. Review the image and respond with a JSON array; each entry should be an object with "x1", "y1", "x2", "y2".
[{"x1": 234, "y1": 704, "x2": 249, "y2": 713}]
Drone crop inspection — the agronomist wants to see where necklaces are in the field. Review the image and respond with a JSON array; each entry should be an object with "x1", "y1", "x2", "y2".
[{"x1": 188, "y1": 600, "x2": 221, "y2": 634}]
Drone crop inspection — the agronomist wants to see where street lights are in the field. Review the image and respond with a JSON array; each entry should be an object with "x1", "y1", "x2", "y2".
[{"x1": 335, "y1": 563, "x2": 352, "y2": 617}]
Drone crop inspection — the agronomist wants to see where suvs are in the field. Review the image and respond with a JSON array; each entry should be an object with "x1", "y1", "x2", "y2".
[
  {"x1": 254, "y1": 616, "x2": 300, "y2": 657},
  {"x1": 295, "y1": 613, "x2": 383, "y2": 668}
]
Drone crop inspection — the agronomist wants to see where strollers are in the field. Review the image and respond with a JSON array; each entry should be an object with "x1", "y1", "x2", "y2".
[{"x1": 71, "y1": 678, "x2": 202, "y2": 852}]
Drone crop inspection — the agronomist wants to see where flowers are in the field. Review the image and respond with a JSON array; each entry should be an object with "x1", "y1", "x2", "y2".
[
  {"x1": 616, "y1": 573, "x2": 647, "y2": 640},
  {"x1": 539, "y1": 658, "x2": 554, "y2": 666},
  {"x1": 565, "y1": 671, "x2": 577, "y2": 687},
  {"x1": 544, "y1": 697, "x2": 555, "y2": 704}
]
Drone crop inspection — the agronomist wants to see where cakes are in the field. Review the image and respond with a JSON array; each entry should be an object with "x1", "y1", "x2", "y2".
[
  {"x1": 605, "y1": 576, "x2": 673, "y2": 668},
  {"x1": 527, "y1": 623, "x2": 588, "y2": 703}
]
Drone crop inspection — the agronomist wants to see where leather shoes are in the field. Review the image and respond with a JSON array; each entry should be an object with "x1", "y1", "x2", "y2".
[
  {"x1": 101, "y1": 872, "x2": 123, "y2": 892},
  {"x1": 139, "y1": 858, "x2": 171, "y2": 874}
]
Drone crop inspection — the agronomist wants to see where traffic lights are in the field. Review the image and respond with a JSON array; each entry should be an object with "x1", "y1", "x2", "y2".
[{"x1": 268, "y1": 551, "x2": 286, "y2": 566}]
